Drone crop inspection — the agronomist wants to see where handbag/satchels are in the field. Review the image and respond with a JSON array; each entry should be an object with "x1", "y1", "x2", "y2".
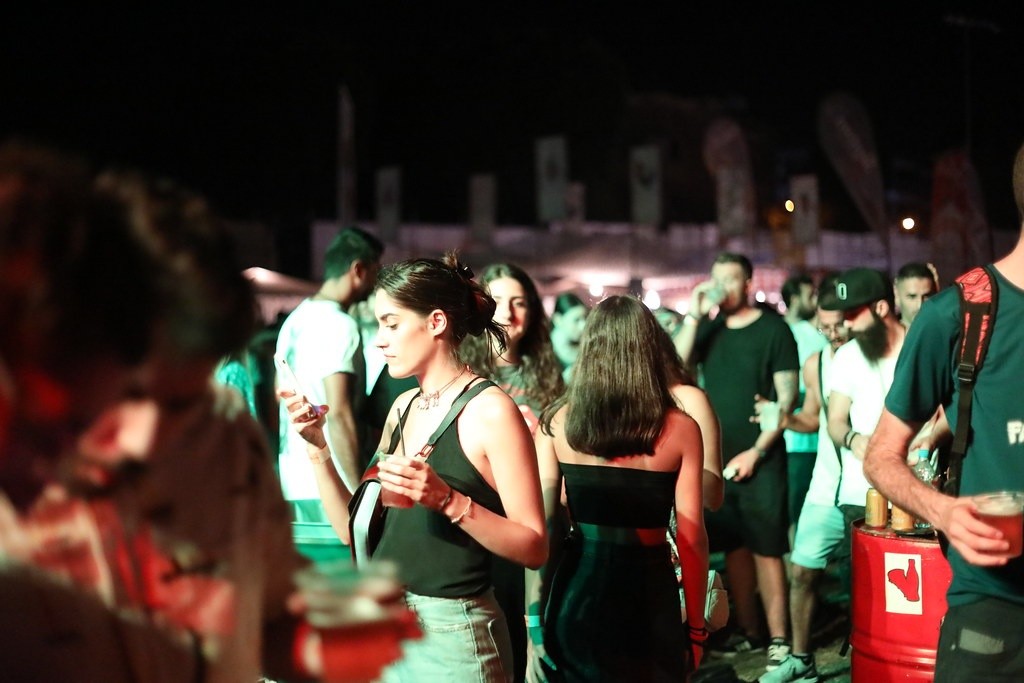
[
  {"x1": 346, "y1": 464, "x2": 387, "y2": 572},
  {"x1": 680, "y1": 569, "x2": 730, "y2": 632}
]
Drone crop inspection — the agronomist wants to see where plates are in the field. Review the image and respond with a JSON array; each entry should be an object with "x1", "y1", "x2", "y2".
[{"x1": 893, "y1": 527, "x2": 935, "y2": 538}]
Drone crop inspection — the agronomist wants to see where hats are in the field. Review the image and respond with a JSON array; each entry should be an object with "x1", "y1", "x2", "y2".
[{"x1": 821, "y1": 268, "x2": 893, "y2": 311}]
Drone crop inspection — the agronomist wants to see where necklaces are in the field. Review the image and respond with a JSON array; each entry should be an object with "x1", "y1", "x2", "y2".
[{"x1": 416, "y1": 363, "x2": 476, "y2": 410}]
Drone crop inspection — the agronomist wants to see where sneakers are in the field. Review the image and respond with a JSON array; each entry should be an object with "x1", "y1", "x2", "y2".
[
  {"x1": 765, "y1": 637, "x2": 792, "y2": 672},
  {"x1": 710, "y1": 626, "x2": 764, "y2": 658},
  {"x1": 758, "y1": 652, "x2": 818, "y2": 683}
]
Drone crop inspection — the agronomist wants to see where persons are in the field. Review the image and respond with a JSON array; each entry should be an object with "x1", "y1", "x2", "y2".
[
  {"x1": 863, "y1": 145, "x2": 1024, "y2": 683},
  {"x1": 212, "y1": 229, "x2": 954, "y2": 683},
  {"x1": 0, "y1": 147, "x2": 296, "y2": 683}
]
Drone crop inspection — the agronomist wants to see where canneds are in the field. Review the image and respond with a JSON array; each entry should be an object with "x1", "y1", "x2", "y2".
[
  {"x1": 891, "y1": 504, "x2": 914, "y2": 532},
  {"x1": 865, "y1": 487, "x2": 889, "y2": 529},
  {"x1": 705, "y1": 286, "x2": 728, "y2": 306}
]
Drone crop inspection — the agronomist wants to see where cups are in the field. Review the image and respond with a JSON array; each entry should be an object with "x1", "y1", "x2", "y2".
[
  {"x1": 968, "y1": 490, "x2": 1024, "y2": 558},
  {"x1": 377, "y1": 453, "x2": 428, "y2": 510},
  {"x1": 707, "y1": 282, "x2": 730, "y2": 304},
  {"x1": 755, "y1": 402, "x2": 780, "y2": 432},
  {"x1": 294, "y1": 559, "x2": 403, "y2": 683}
]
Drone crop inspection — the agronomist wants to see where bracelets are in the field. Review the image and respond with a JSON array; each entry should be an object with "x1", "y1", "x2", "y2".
[
  {"x1": 751, "y1": 443, "x2": 766, "y2": 457},
  {"x1": 307, "y1": 443, "x2": 332, "y2": 464},
  {"x1": 451, "y1": 496, "x2": 471, "y2": 523},
  {"x1": 688, "y1": 625, "x2": 706, "y2": 631},
  {"x1": 689, "y1": 633, "x2": 708, "y2": 640},
  {"x1": 844, "y1": 430, "x2": 852, "y2": 449},
  {"x1": 690, "y1": 638, "x2": 707, "y2": 646},
  {"x1": 848, "y1": 432, "x2": 861, "y2": 449},
  {"x1": 438, "y1": 486, "x2": 453, "y2": 514},
  {"x1": 524, "y1": 616, "x2": 544, "y2": 628}
]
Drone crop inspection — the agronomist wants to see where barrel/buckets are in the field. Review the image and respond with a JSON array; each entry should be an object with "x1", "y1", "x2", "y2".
[{"x1": 850, "y1": 516, "x2": 954, "y2": 683}]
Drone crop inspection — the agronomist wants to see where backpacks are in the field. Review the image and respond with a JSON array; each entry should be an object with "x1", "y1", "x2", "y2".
[{"x1": 935, "y1": 265, "x2": 1000, "y2": 559}]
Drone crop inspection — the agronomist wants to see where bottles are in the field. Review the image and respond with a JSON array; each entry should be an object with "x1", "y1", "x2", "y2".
[{"x1": 911, "y1": 449, "x2": 935, "y2": 529}]
